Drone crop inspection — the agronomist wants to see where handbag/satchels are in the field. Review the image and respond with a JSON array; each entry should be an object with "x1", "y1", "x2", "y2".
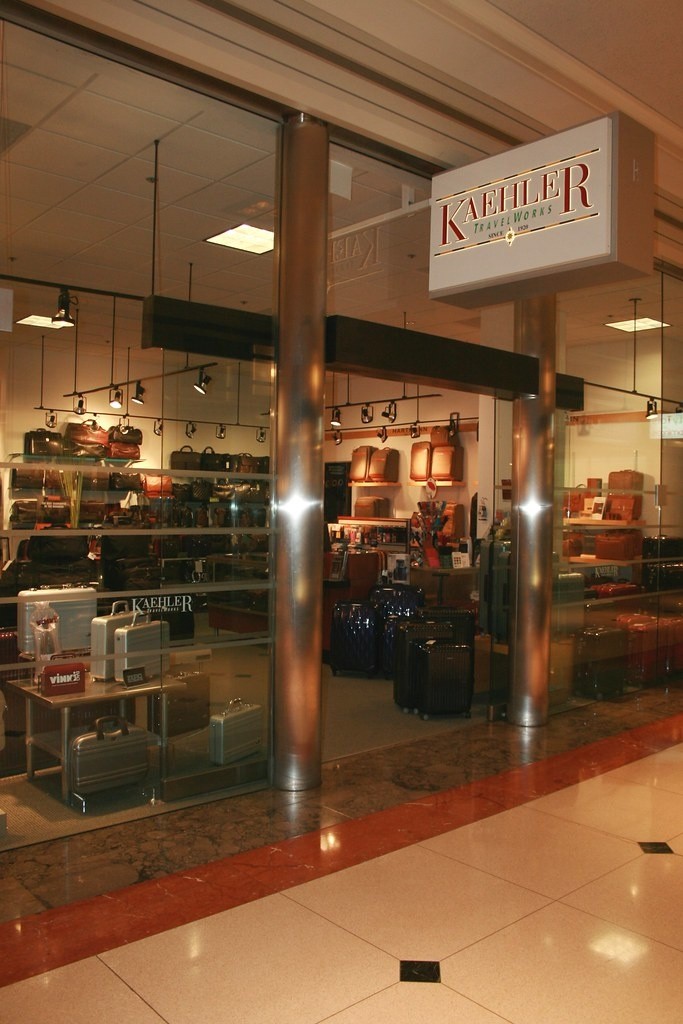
[
  {"x1": 0, "y1": 469, "x2": 271, "y2": 528},
  {"x1": 16, "y1": 534, "x2": 269, "y2": 592},
  {"x1": 23, "y1": 419, "x2": 142, "y2": 459},
  {"x1": 205, "y1": 588, "x2": 270, "y2": 613},
  {"x1": 171, "y1": 445, "x2": 271, "y2": 473}
]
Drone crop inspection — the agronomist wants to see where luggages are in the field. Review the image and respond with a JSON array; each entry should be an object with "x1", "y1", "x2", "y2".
[
  {"x1": 354, "y1": 496, "x2": 389, "y2": 517},
  {"x1": 353, "y1": 445, "x2": 399, "y2": 480},
  {"x1": 328, "y1": 521, "x2": 524, "y2": 719},
  {"x1": 552, "y1": 534, "x2": 683, "y2": 700},
  {"x1": 436, "y1": 502, "x2": 466, "y2": 542},
  {"x1": 410, "y1": 427, "x2": 463, "y2": 482}
]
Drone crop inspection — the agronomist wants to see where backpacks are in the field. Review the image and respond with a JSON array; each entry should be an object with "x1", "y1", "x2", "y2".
[{"x1": 563, "y1": 469, "x2": 643, "y2": 560}]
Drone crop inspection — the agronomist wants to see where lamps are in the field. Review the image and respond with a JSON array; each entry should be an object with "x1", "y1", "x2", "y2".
[
  {"x1": 581, "y1": 298, "x2": 683, "y2": 419},
  {"x1": 35, "y1": 137, "x2": 480, "y2": 444}
]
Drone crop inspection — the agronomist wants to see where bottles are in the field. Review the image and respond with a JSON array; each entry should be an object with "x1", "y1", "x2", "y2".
[{"x1": 31, "y1": 601, "x2": 62, "y2": 685}]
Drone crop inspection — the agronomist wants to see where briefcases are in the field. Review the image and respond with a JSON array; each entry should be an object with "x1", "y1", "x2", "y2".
[
  {"x1": 69, "y1": 716, "x2": 148, "y2": 793},
  {"x1": 148, "y1": 672, "x2": 210, "y2": 738},
  {"x1": 91, "y1": 601, "x2": 170, "y2": 681},
  {"x1": 17, "y1": 582, "x2": 98, "y2": 653},
  {"x1": 209, "y1": 698, "x2": 263, "y2": 766}
]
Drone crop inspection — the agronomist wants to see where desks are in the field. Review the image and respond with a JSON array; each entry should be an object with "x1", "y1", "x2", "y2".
[{"x1": 6, "y1": 675, "x2": 187, "y2": 806}]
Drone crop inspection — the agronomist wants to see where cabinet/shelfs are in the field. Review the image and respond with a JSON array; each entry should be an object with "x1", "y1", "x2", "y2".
[
  {"x1": 1, "y1": 462, "x2": 271, "y2": 607},
  {"x1": 323, "y1": 480, "x2": 468, "y2": 649},
  {"x1": 562, "y1": 483, "x2": 648, "y2": 568}
]
